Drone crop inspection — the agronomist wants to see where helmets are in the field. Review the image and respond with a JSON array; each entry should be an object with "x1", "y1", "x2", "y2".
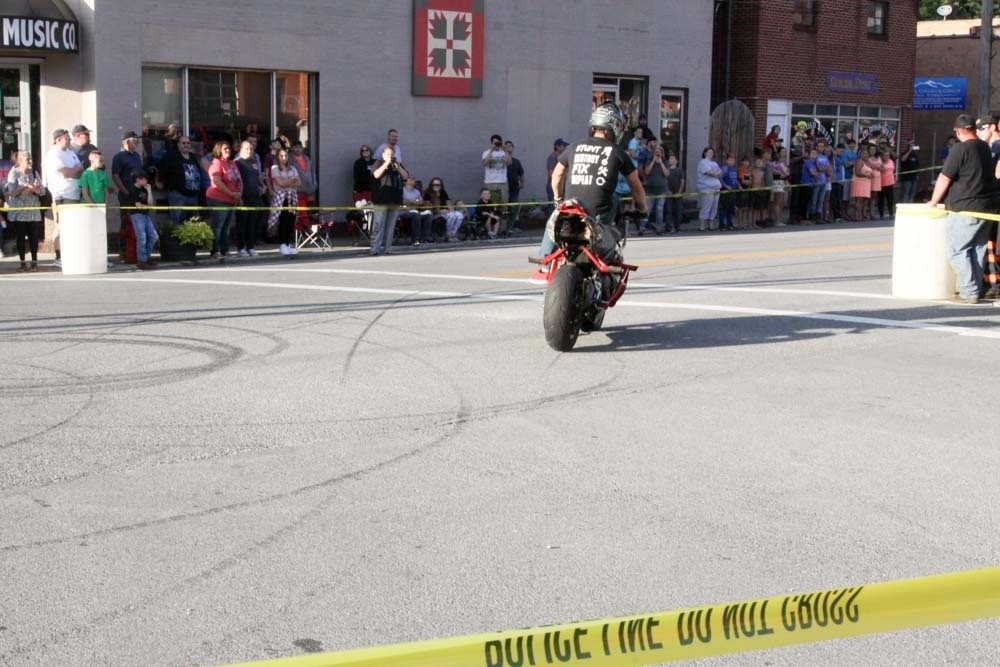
[{"x1": 588, "y1": 101, "x2": 626, "y2": 145}]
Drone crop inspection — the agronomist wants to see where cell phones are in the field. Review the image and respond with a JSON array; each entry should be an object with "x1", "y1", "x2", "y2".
[
  {"x1": 912, "y1": 146, "x2": 920, "y2": 150},
  {"x1": 495, "y1": 142, "x2": 502, "y2": 146}
]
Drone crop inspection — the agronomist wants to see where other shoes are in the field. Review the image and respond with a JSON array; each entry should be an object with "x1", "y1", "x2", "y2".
[
  {"x1": 950, "y1": 294, "x2": 977, "y2": 304},
  {"x1": 446, "y1": 238, "x2": 454, "y2": 242},
  {"x1": 629, "y1": 205, "x2": 896, "y2": 232},
  {"x1": 984, "y1": 288, "x2": 1000, "y2": 299},
  {"x1": 453, "y1": 237, "x2": 460, "y2": 242},
  {"x1": 16, "y1": 244, "x2": 297, "y2": 272}
]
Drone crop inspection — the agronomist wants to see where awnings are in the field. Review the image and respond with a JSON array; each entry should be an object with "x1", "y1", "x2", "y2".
[{"x1": 0, "y1": 0, "x2": 81, "y2": 57}]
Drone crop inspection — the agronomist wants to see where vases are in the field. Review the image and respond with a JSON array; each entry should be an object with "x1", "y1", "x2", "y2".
[{"x1": 158, "y1": 236, "x2": 181, "y2": 262}]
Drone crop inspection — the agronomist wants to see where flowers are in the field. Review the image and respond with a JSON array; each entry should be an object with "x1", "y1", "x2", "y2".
[{"x1": 153, "y1": 215, "x2": 180, "y2": 236}]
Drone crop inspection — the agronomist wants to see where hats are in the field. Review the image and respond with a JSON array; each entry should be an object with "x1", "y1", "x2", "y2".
[
  {"x1": 294, "y1": 141, "x2": 302, "y2": 146},
  {"x1": 54, "y1": 129, "x2": 68, "y2": 144},
  {"x1": 72, "y1": 125, "x2": 91, "y2": 136},
  {"x1": 131, "y1": 168, "x2": 149, "y2": 179},
  {"x1": 953, "y1": 115, "x2": 975, "y2": 131},
  {"x1": 976, "y1": 116, "x2": 996, "y2": 128},
  {"x1": 122, "y1": 130, "x2": 142, "y2": 140},
  {"x1": 554, "y1": 139, "x2": 570, "y2": 145}
]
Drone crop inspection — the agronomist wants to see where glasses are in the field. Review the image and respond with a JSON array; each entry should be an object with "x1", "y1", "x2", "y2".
[
  {"x1": 978, "y1": 125, "x2": 989, "y2": 130},
  {"x1": 432, "y1": 184, "x2": 441, "y2": 186},
  {"x1": 362, "y1": 149, "x2": 369, "y2": 152}
]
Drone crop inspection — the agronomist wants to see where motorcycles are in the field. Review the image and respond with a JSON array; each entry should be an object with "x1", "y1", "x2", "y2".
[{"x1": 529, "y1": 199, "x2": 647, "y2": 350}]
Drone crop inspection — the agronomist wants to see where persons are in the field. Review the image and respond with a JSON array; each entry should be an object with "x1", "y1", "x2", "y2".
[
  {"x1": 127, "y1": 166, "x2": 159, "y2": 268},
  {"x1": 545, "y1": 137, "x2": 570, "y2": 219},
  {"x1": 695, "y1": 147, "x2": 722, "y2": 231},
  {"x1": 45, "y1": 125, "x2": 110, "y2": 268},
  {"x1": 352, "y1": 128, "x2": 475, "y2": 256},
  {"x1": 6, "y1": 149, "x2": 47, "y2": 272},
  {"x1": 925, "y1": 108, "x2": 1000, "y2": 305},
  {"x1": 791, "y1": 119, "x2": 921, "y2": 225},
  {"x1": 719, "y1": 123, "x2": 791, "y2": 231},
  {"x1": 612, "y1": 113, "x2": 685, "y2": 236},
  {"x1": 475, "y1": 189, "x2": 500, "y2": 241},
  {"x1": 538, "y1": 102, "x2": 648, "y2": 286},
  {"x1": 109, "y1": 131, "x2": 144, "y2": 261},
  {"x1": 480, "y1": 135, "x2": 525, "y2": 235},
  {"x1": 156, "y1": 134, "x2": 319, "y2": 260}
]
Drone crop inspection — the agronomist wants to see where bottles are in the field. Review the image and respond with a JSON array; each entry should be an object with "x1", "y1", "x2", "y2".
[{"x1": 34, "y1": 178, "x2": 40, "y2": 194}]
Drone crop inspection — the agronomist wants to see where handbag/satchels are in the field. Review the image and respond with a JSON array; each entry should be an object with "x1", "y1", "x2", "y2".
[{"x1": 39, "y1": 188, "x2": 52, "y2": 212}]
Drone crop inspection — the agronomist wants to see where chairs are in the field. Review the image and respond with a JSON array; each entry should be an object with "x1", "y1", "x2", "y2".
[
  {"x1": 391, "y1": 180, "x2": 432, "y2": 245},
  {"x1": 295, "y1": 191, "x2": 335, "y2": 251},
  {"x1": 351, "y1": 190, "x2": 401, "y2": 246}
]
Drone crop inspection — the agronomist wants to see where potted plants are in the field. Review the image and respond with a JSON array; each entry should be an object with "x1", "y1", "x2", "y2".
[{"x1": 172, "y1": 216, "x2": 216, "y2": 262}]
